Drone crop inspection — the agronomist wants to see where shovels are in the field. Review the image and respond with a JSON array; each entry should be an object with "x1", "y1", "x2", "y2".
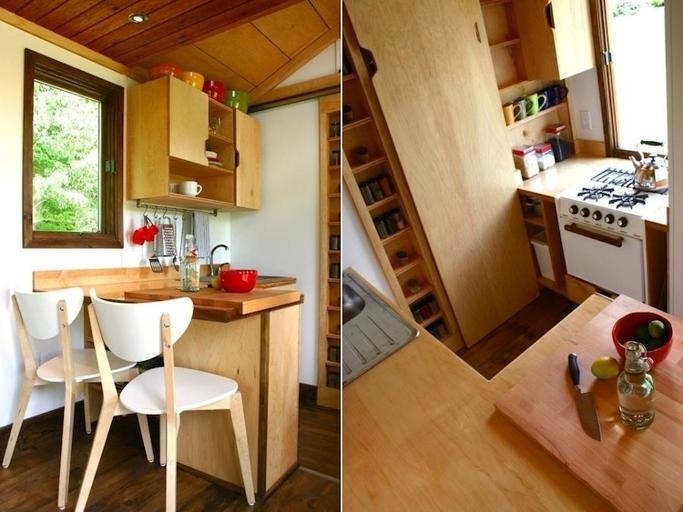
[{"x1": 149, "y1": 216, "x2": 163, "y2": 272}]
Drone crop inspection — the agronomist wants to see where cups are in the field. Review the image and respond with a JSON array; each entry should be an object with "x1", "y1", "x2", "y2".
[
  {"x1": 503, "y1": 81, "x2": 569, "y2": 125},
  {"x1": 179, "y1": 181, "x2": 202, "y2": 197}
]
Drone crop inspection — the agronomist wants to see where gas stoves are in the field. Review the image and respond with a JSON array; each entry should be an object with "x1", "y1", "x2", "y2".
[{"x1": 555, "y1": 158, "x2": 668, "y2": 239}]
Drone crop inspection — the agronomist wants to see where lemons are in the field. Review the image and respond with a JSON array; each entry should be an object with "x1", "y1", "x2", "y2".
[
  {"x1": 212, "y1": 276, "x2": 223, "y2": 290},
  {"x1": 592, "y1": 357, "x2": 620, "y2": 380},
  {"x1": 637, "y1": 320, "x2": 665, "y2": 351}
]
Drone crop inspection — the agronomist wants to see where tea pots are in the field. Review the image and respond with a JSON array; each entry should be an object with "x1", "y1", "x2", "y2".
[{"x1": 629, "y1": 140, "x2": 669, "y2": 191}]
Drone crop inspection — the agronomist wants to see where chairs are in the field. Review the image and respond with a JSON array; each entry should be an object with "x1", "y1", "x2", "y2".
[
  {"x1": 2, "y1": 288, "x2": 156, "y2": 509},
  {"x1": 75, "y1": 288, "x2": 256, "y2": 512}
]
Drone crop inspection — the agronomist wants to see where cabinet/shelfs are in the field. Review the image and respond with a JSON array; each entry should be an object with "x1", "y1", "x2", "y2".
[
  {"x1": 513, "y1": 0, "x2": 594, "y2": 81},
  {"x1": 344, "y1": 0, "x2": 577, "y2": 350},
  {"x1": 128, "y1": 77, "x2": 263, "y2": 212},
  {"x1": 317, "y1": 93, "x2": 341, "y2": 412},
  {"x1": 645, "y1": 219, "x2": 670, "y2": 315},
  {"x1": 343, "y1": 5, "x2": 466, "y2": 354},
  {"x1": 518, "y1": 190, "x2": 565, "y2": 300}
]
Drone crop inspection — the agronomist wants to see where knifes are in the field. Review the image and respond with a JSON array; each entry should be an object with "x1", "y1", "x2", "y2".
[{"x1": 568, "y1": 353, "x2": 601, "y2": 446}]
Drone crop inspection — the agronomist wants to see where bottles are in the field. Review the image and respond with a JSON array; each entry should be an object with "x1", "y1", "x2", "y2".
[
  {"x1": 358, "y1": 171, "x2": 406, "y2": 239},
  {"x1": 330, "y1": 235, "x2": 340, "y2": 250},
  {"x1": 327, "y1": 372, "x2": 340, "y2": 388},
  {"x1": 328, "y1": 345, "x2": 339, "y2": 361},
  {"x1": 331, "y1": 150, "x2": 340, "y2": 165},
  {"x1": 182, "y1": 234, "x2": 201, "y2": 292},
  {"x1": 413, "y1": 300, "x2": 448, "y2": 339},
  {"x1": 617, "y1": 341, "x2": 655, "y2": 431},
  {"x1": 330, "y1": 121, "x2": 340, "y2": 139},
  {"x1": 330, "y1": 264, "x2": 340, "y2": 278},
  {"x1": 511, "y1": 123, "x2": 571, "y2": 178}
]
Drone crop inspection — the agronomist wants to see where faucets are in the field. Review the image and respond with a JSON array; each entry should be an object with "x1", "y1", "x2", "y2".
[{"x1": 210, "y1": 244, "x2": 229, "y2": 280}]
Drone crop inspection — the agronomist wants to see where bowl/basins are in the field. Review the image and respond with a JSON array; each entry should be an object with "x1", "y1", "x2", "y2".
[
  {"x1": 218, "y1": 270, "x2": 257, "y2": 293},
  {"x1": 612, "y1": 311, "x2": 674, "y2": 371},
  {"x1": 148, "y1": 61, "x2": 249, "y2": 113}
]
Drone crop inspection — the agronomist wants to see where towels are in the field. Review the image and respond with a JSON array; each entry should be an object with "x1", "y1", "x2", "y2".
[{"x1": 192, "y1": 213, "x2": 211, "y2": 259}]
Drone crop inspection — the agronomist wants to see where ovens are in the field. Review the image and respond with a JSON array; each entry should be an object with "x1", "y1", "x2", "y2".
[{"x1": 557, "y1": 219, "x2": 648, "y2": 304}]
